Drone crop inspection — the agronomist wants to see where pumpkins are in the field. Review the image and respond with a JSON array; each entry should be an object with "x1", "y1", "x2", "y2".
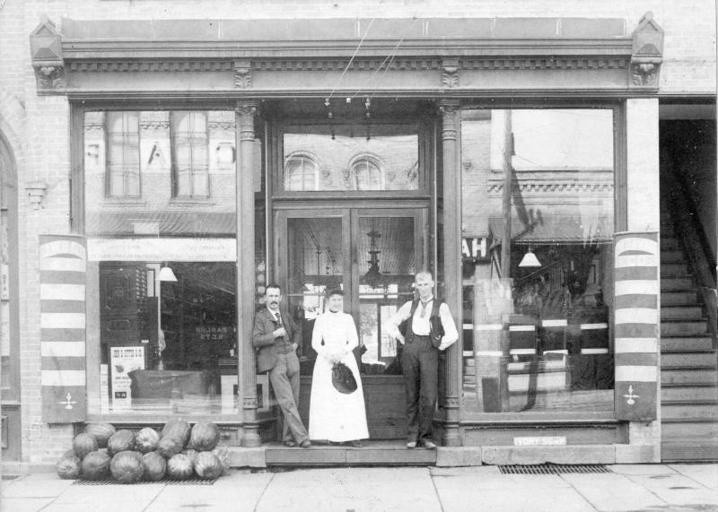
[{"x1": 56, "y1": 417, "x2": 223, "y2": 481}]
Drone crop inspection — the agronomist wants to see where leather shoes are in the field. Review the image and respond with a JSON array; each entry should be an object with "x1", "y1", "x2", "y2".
[
  {"x1": 284, "y1": 441, "x2": 295, "y2": 446},
  {"x1": 420, "y1": 441, "x2": 436, "y2": 450},
  {"x1": 406, "y1": 442, "x2": 417, "y2": 449},
  {"x1": 300, "y1": 440, "x2": 311, "y2": 447}
]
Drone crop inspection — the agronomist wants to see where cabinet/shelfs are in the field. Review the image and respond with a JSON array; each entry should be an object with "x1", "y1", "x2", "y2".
[{"x1": 161, "y1": 273, "x2": 235, "y2": 370}]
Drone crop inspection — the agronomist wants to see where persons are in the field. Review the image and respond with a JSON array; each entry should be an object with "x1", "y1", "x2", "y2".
[
  {"x1": 252, "y1": 283, "x2": 310, "y2": 448},
  {"x1": 388, "y1": 270, "x2": 460, "y2": 450},
  {"x1": 306, "y1": 289, "x2": 370, "y2": 448}
]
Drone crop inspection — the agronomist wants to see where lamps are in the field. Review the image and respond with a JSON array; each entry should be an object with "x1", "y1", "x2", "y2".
[
  {"x1": 518, "y1": 246, "x2": 542, "y2": 267},
  {"x1": 155, "y1": 261, "x2": 178, "y2": 282}
]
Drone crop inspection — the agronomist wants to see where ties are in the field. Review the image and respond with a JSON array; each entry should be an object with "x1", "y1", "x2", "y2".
[{"x1": 275, "y1": 312, "x2": 282, "y2": 328}]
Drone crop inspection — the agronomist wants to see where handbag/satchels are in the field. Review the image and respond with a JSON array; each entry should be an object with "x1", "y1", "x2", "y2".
[{"x1": 331, "y1": 362, "x2": 357, "y2": 395}]
